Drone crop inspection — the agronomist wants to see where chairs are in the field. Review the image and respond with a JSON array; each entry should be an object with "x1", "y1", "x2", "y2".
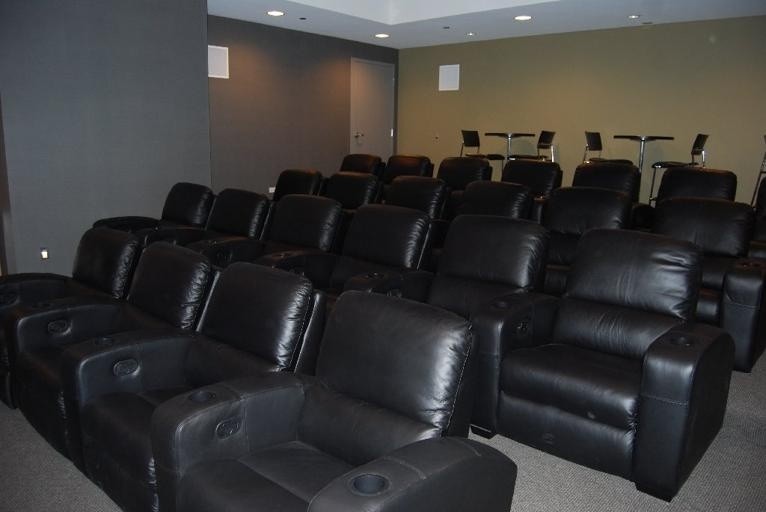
[
  {"x1": 513, "y1": 130, "x2": 556, "y2": 165},
  {"x1": 581, "y1": 132, "x2": 608, "y2": 162},
  {"x1": 647, "y1": 133, "x2": 708, "y2": 205},
  {"x1": 458, "y1": 129, "x2": 504, "y2": 172}
]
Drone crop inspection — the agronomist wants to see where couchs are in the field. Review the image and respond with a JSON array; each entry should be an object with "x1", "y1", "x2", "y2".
[
  {"x1": 60, "y1": 258, "x2": 332, "y2": 512},
  {"x1": 533, "y1": 184, "x2": 636, "y2": 287},
  {"x1": 455, "y1": 180, "x2": 531, "y2": 221},
  {"x1": 383, "y1": 154, "x2": 436, "y2": 179},
  {"x1": 322, "y1": 169, "x2": 378, "y2": 207},
  {"x1": 5, "y1": 226, "x2": 142, "y2": 414},
  {"x1": 280, "y1": 201, "x2": 439, "y2": 311},
  {"x1": 151, "y1": 288, "x2": 521, "y2": 512},
  {"x1": 641, "y1": 193, "x2": 765, "y2": 376},
  {"x1": 380, "y1": 175, "x2": 449, "y2": 219},
  {"x1": 358, "y1": 211, "x2": 558, "y2": 441},
  {"x1": 473, "y1": 224, "x2": 738, "y2": 505},
  {"x1": 500, "y1": 156, "x2": 564, "y2": 204},
  {"x1": 268, "y1": 167, "x2": 322, "y2": 200},
  {"x1": 338, "y1": 154, "x2": 382, "y2": 177},
  {"x1": 569, "y1": 156, "x2": 643, "y2": 203},
  {"x1": 436, "y1": 155, "x2": 494, "y2": 188},
  {"x1": 91, "y1": 178, "x2": 346, "y2": 276},
  {"x1": 655, "y1": 164, "x2": 740, "y2": 202},
  {"x1": 7, "y1": 239, "x2": 225, "y2": 469}
]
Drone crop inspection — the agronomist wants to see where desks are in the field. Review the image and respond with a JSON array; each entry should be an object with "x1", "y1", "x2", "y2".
[
  {"x1": 613, "y1": 134, "x2": 673, "y2": 182},
  {"x1": 485, "y1": 132, "x2": 535, "y2": 163}
]
